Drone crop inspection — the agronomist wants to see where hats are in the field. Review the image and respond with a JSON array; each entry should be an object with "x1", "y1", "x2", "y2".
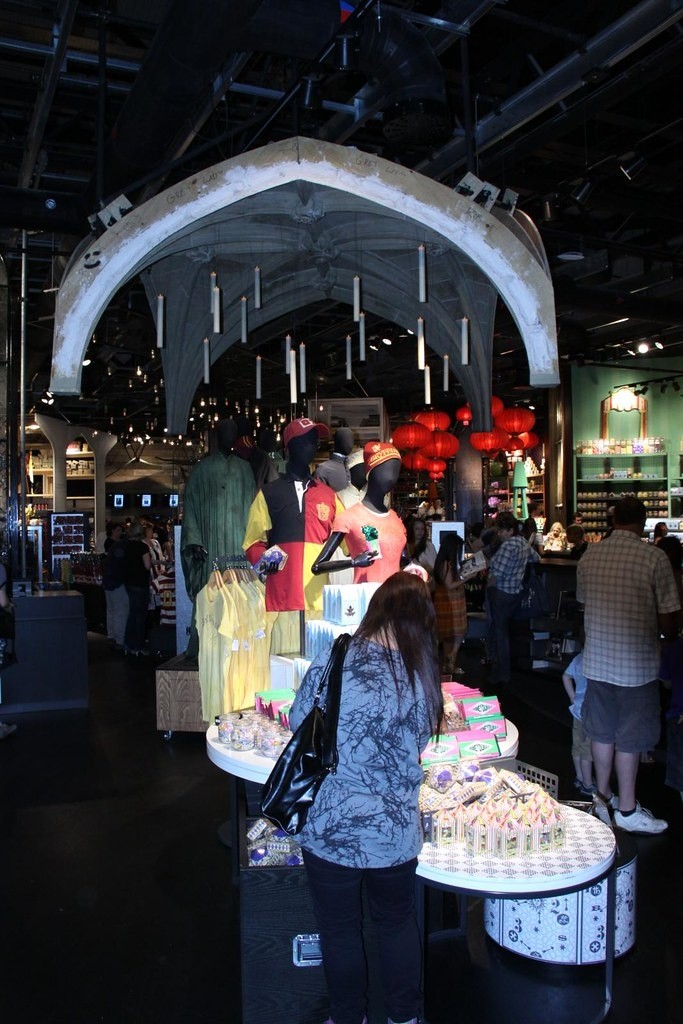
[
  {"x1": 283, "y1": 417, "x2": 331, "y2": 452},
  {"x1": 363, "y1": 441, "x2": 402, "y2": 481}
]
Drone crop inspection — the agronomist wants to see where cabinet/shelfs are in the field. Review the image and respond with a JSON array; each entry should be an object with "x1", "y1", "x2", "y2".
[
  {"x1": 307, "y1": 396, "x2": 397, "y2": 457},
  {"x1": 23, "y1": 443, "x2": 53, "y2": 496},
  {"x1": 572, "y1": 452, "x2": 673, "y2": 539},
  {"x1": 64, "y1": 451, "x2": 95, "y2": 479},
  {"x1": 18, "y1": 437, "x2": 95, "y2": 582},
  {"x1": 487, "y1": 473, "x2": 546, "y2": 527}
]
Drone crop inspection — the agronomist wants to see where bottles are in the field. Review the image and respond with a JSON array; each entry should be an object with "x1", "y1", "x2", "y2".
[
  {"x1": 576, "y1": 437, "x2": 665, "y2": 455},
  {"x1": 51, "y1": 549, "x2": 104, "y2": 584},
  {"x1": 219, "y1": 710, "x2": 294, "y2": 759}
]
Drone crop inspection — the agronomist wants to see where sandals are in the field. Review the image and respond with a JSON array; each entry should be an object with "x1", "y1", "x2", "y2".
[{"x1": 442, "y1": 656, "x2": 466, "y2": 675}]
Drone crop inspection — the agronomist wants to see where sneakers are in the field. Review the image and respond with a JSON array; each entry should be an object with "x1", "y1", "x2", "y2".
[
  {"x1": 573, "y1": 776, "x2": 583, "y2": 790},
  {"x1": 612, "y1": 807, "x2": 669, "y2": 836},
  {"x1": 580, "y1": 783, "x2": 597, "y2": 796},
  {"x1": 605, "y1": 792, "x2": 618, "y2": 811}
]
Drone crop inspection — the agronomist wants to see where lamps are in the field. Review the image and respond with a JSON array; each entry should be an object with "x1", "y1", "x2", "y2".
[
  {"x1": 334, "y1": 28, "x2": 363, "y2": 72},
  {"x1": 670, "y1": 379, "x2": 680, "y2": 391},
  {"x1": 617, "y1": 149, "x2": 649, "y2": 182},
  {"x1": 298, "y1": 69, "x2": 327, "y2": 112},
  {"x1": 540, "y1": 192, "x2": 560, "y2": 222},
  {"x1": 634, "y1": 384, "x2": 650, "y2": 396},
  {"x1": 568, "y1": 176, "x2": 599, "y2": 204},
  {"x1": 40, "y1": 387, "x2": 55, "y2": 406},
  {"x1": 636, "y1": 340, "x2": 651, "y2": 354},
  {"x1": 157, "y1": 244, "x2": 471, "y2": 406},
  {"x1": 625, "y1": 347, "x2": 638, "y2": 357},
  {"x1": 654, "y1": 334, "x2": 670, "y2": 350},
  {"x1": 660, "y1": 381, "x2": 669, "y2": 393}
]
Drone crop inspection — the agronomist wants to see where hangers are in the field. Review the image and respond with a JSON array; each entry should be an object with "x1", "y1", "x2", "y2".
[{"x1": 205, "y1": 551, "x2": 255, "y2": 592}]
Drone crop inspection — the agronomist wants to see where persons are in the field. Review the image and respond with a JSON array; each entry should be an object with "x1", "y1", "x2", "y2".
[
  {"x1": 242, "y1": 417, "x2": 350, "y2": 611},
  {"x1": 289, "y1": 571, "x2": 444, "y2": 1024},
  {"x1": 311, "y1": 441, "x2": 428, "y2": 583},
  {"x1": 180, "y1": 418, "x2": 257, "y2": 663},
  {"x1": 562, "y1": 625, "x2": 597, "y2": 795},
  {"x1": 338, "y1": 448, "x2": 389, "y2": 508},
  {"x1": 545, "y1": 512, "x2": 588, "y2": 560},
  {"x1": 403, "y1": 512, "x2": 543, "y2": 687},
  {"x1": 0, "y1": 564, "x2": 17, "y2": 738},
  {"x1": 576, "y1": 497, "x2": 683, "y2": 834},
  {"x1": 313, "y1": 428, "x2": 353, "y2": 490},
  {"x1": 102, "y1": 521, "x2": 172, "y2": 657}
]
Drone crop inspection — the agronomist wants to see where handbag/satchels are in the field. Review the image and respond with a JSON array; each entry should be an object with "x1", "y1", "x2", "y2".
[{"x1": 258, "y1": 706, "x2": 340, "y2": 841}]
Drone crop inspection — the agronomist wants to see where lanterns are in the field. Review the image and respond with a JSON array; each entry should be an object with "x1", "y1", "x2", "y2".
[{"x1": 392, "y1": 395, "x2": 539, "y2": 483}]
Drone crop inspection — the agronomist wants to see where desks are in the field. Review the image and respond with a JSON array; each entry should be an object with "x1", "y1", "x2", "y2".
[
  {"x1": 414, "y1": 803, "x2": 620, "y2": 1024},
  {"x1": 205, "y1": 711, "x2": 294, "y2": 884}
]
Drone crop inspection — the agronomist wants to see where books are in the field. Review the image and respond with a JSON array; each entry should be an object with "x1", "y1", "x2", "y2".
[{"x1": 459, "y1": 550, "x2": 486, "y2": 578}]
